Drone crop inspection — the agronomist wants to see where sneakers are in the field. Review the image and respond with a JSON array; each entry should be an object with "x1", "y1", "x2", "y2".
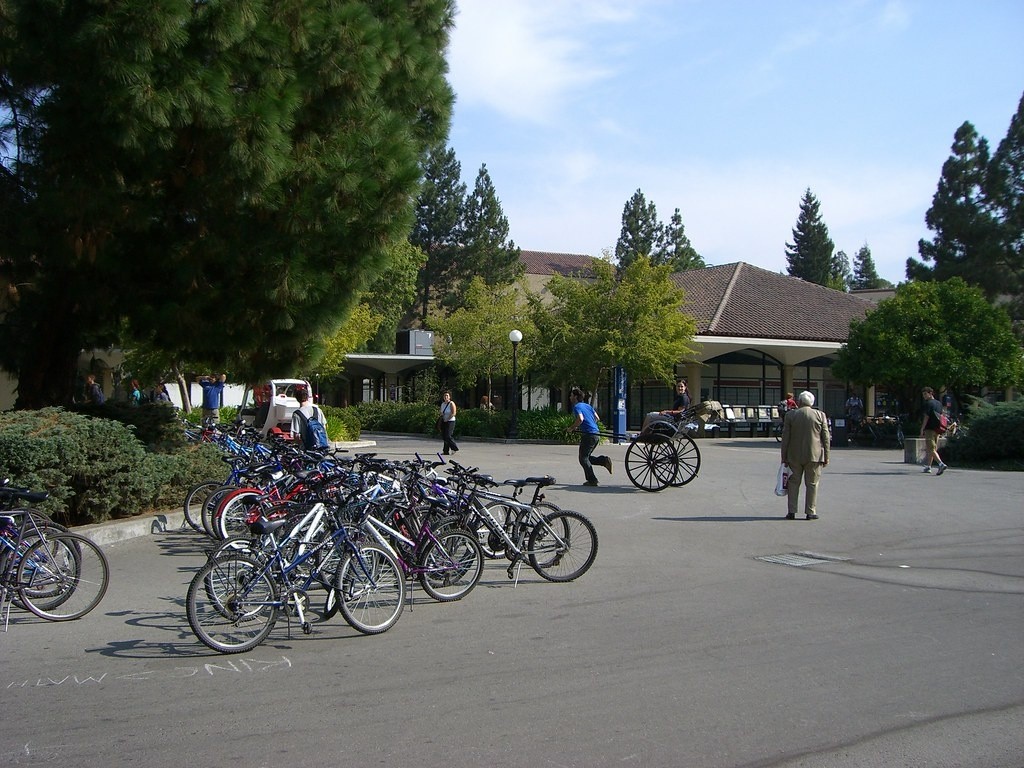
[
  {"x1": 923, "y1": 466, "x2": 932, "y2": 474},
  {"x1": 936, "y1": 464, "x2": 948, "y2": 475}
]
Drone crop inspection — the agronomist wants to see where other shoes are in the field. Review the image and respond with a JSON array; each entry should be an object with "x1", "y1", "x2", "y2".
[
  {"x1": 441, "y1": 450, "x2": 450, "y2": 455},
  {"x1": 806, "y1": 515, "x2": 821, "y2": 520},
  {"x1": 451, "y1": 450, "x2": 459, "y2": 454},
  {"x1": 786, "y1": 513, "x2": 796, "y2": 519},
  {"x1": 605, "y1": 457, "x2": 614, "y2": 474},
  {"x1": 584, "y1": 479, "x2": 598, "y2": 486}
]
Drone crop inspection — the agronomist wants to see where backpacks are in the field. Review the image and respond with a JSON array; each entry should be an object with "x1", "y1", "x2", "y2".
[
  {"x1": 778, "y1": 400, "x2": 788, "y2": 413},
  {"x1": 933, "y1": 408, "x2": 949, "y2": 434},
  {"x1": 294, "y1": 406, "x2": 330, "y2": 450}
]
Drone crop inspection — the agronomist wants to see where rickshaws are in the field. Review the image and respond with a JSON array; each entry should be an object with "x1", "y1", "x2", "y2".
[{"x1": 564, "y1": 402, "x2": 725, "y2": 494}]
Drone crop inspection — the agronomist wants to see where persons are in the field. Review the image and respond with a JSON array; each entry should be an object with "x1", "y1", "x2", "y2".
[
  {"x1": 567, "y1": 388, "x2": 612, "y2": 486},
  {"x1": 846, "y1": 393, "x2": 863, "y2": 416},
  {"x1": 78, "y1": 375, "x2": 105, "y2": 404},
  {"x1": 781, "y1": 391, "x2": 829, "y2": 520},
  {"x1": 440, "y1": 391, "x2": 459, "y2": 455},
  {"x1": 642, "y1": 379, "x2": 691, "y2": 433},
  {"x1": 786, "y1": 393, "x2": 798, "y2": 411},
  {"x1": 920, "y1": 387, "x2": 947, "y2": 475},
  {"x1": 153, "y1": 376, "x2": 170, "y2": 403},
  {"x1": 195, "y1": 372, "x2": 226, "y2": 428},
  {"x1": 292, "y1": 389, "x2": 327, "y2": 449},
  {"x1": 127, "y1": 379, "x2": 140, "y2": 403}
]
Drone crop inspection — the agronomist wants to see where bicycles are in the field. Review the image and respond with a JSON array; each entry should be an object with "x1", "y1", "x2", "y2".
[
  {"x1": 177, "y1": 418, "x2": 600, "y2": 618},
  {"x1": 853, "y1": 416, "x2": 906, "y2": 446},
  {"x1": 0, "y1": 480, "x2": 111, "y2": 633},
  {"x1": 180, "y1": 485, "x2": 406, "y2": 654},
  {"x1": 775, "y1": 418, "x2": 784, "y2": 443}
]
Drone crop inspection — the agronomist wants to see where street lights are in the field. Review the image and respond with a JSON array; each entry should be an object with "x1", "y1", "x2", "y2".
[{"x1": 509, "y1": 330, "x2": 523, "y2": 440}]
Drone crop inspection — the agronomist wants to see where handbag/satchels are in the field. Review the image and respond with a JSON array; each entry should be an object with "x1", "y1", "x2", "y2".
[
  {"x1": 436, "y1": 416, "x2": 443, "y2": 431},
  {"x1": 775, "y1": 463, "x2": 793, "y2": 496}
]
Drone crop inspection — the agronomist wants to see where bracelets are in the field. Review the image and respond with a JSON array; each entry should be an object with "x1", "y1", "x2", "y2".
[{"x1": 672, "y1": 410, "x2": 674, "y2": 413}]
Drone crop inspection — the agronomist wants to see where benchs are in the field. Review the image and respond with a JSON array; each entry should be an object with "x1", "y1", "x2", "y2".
[{"x1": 646, "y1": 401, "x2": 722, "y2": 436}]
